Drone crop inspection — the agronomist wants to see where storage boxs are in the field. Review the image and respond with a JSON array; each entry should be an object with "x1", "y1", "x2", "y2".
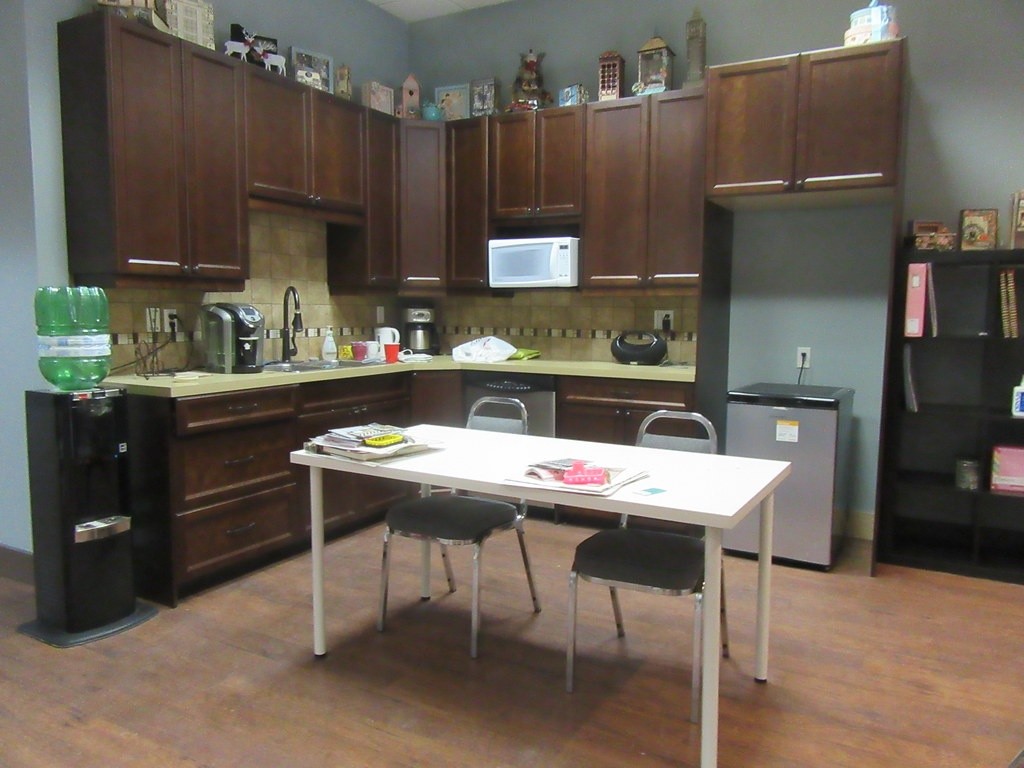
[
  {"x1": 359, "y1": 83, "x2": 399, "y2": 115},
  {"x1": 903, "y1": 263, "x2": 928, "y2": 336},
  {"x1": 959, "y1": 208, "x2": 1000, "y2": 254},
  {"x1": 292, "y1": 46, "x2": 337, "y2": 94},
  {"x1": 989, "y1": 445, "x2": 1024, "y2": 494},
  {"x1": 231, "y1": 23, "x2": 280, "y2": 74},
  {"x1": 472, "y1": 78, "x2": 498, "y2": 116}
]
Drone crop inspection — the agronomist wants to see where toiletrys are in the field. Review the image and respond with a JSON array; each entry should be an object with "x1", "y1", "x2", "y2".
[{"x1": 321, "y1": 325, "x2": 338, "y2": 361}]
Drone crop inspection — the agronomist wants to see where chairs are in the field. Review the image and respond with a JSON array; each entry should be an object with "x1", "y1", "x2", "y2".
[
  {"x1": 564, "y1": 410, "x2": 732, "y2": 724},
  {"x1": 379, "y1": 398, "x2": 538, "y2": 665}
]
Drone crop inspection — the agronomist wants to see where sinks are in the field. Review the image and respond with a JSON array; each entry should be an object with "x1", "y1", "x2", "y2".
[{"x1": 264, "y1": 359, "x2": 385, "y2": 372}]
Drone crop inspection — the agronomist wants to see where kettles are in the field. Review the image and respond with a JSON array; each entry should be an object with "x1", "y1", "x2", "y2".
[{"x1": 374, "y1": 322, "x2": 400, "y2": 363}]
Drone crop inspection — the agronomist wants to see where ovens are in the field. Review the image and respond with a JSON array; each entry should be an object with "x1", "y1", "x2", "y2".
[{"x1": 463, "y1": 370, "x2": 557, "y2": 519}]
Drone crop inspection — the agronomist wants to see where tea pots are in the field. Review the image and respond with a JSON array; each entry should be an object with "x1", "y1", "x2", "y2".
[{"x1": 420, "y1": 101, "x2": 448, "y2": 121}]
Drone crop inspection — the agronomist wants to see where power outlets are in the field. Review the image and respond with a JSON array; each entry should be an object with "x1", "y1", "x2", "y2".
[
  {"x1": 164, "y1": 310, "x2": 178, "y2": 331},
  {"x1": 146, "y1": 308, "x2": 161, "y2": 333},
  {"x1": 653, "y1": 310, "x2": 674, "y2": 330},
  {"x1": 796, "y1": 345, "x2": 812, "y2": 369},
  {"x1": 377, "y1": 305, "x2": 385, "y2": 324},
  {"x1": 325, "y1": 100, "x2": 398, "y2": 286}
]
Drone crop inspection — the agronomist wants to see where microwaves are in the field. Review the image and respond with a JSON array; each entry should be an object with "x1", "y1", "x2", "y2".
[{"x1": 487, "y1": 236, "x2": 579, "y2": 290}]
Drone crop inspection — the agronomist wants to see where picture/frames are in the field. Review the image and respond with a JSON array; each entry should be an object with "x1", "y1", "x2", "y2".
[{"x1": 434, "y1": 83, "x2": 469, "y2": 122}]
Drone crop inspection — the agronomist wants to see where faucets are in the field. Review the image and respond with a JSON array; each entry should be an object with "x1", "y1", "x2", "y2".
[{"x1": 282, "y1": 286, "x2": 304, "y2": 363}]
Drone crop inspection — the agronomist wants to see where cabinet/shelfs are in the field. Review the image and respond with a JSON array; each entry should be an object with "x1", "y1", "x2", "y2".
[
  {"x1": 449, "y1": 116, "x2": 497, "y2": 291},
  {"x1": 178, "y1": 385, "x2": 300, "y2": 584},
  {"x1": 296, "y1": 372, "x2": 412, "y2": 541},
  {"x1": 704, "y1": 36, "x2": 912, "y2": 213},
  {"x1": 561, "y1": 380, "x2": 689, "y2": 539},
  {"x1": 56, "y1": 9, "x2": 243, "y2": 276},
  {"x1": 873, "y1": 237, "x2": 1024, "y2": 587},
  {"x1": 397, "y1": 115, "x2": 448, "y2": 289},
  {"x1": 582, "y1": 92, "x2": 733, "y2": 285},
  {"x1": 492, "y1": 106, "x2": 587, "y2": 218}
]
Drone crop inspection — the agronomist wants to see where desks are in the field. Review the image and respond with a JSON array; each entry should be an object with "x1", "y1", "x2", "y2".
[{"x1": 292, "y1": 422, "x2": 797, "y2": 768}]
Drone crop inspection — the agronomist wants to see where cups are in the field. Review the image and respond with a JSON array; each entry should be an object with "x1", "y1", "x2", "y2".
[
  {"x1": 239, "y1": 337, "x2": 259, "y2": 365},
  {"x1": 956, "y1": 451, "x2": 982, "y2": 491},
  {"x1": 337, "y1": 340, "x2": 400, "y2": 364}
]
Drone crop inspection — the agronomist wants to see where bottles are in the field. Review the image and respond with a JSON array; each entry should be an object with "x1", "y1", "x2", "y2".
[{"x1": 34, "y1": 286, "x2": 112, "y2": 392}]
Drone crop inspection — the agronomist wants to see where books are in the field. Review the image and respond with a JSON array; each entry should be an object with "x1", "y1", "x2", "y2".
[
  {"x1": 958, "y1": 208, "x2": 998, "y2": 251},
  {"x1": 1011, "y1": 193, "x2": 1024, "y2": 250},
  {"x1": 1000, "y1": 272, "x2": 1018, "y2": 338},
  {"x1": 927, "y1": 262, "x2": 937, "y2": 338}
]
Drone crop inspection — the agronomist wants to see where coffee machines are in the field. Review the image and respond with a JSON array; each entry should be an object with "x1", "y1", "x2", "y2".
[
  {"x1": 200, "y1": 302, "x2": 265, "y2": 373},
  {"x1": 402, "y1": 299, "x2": 439, "y2": 356}
]
